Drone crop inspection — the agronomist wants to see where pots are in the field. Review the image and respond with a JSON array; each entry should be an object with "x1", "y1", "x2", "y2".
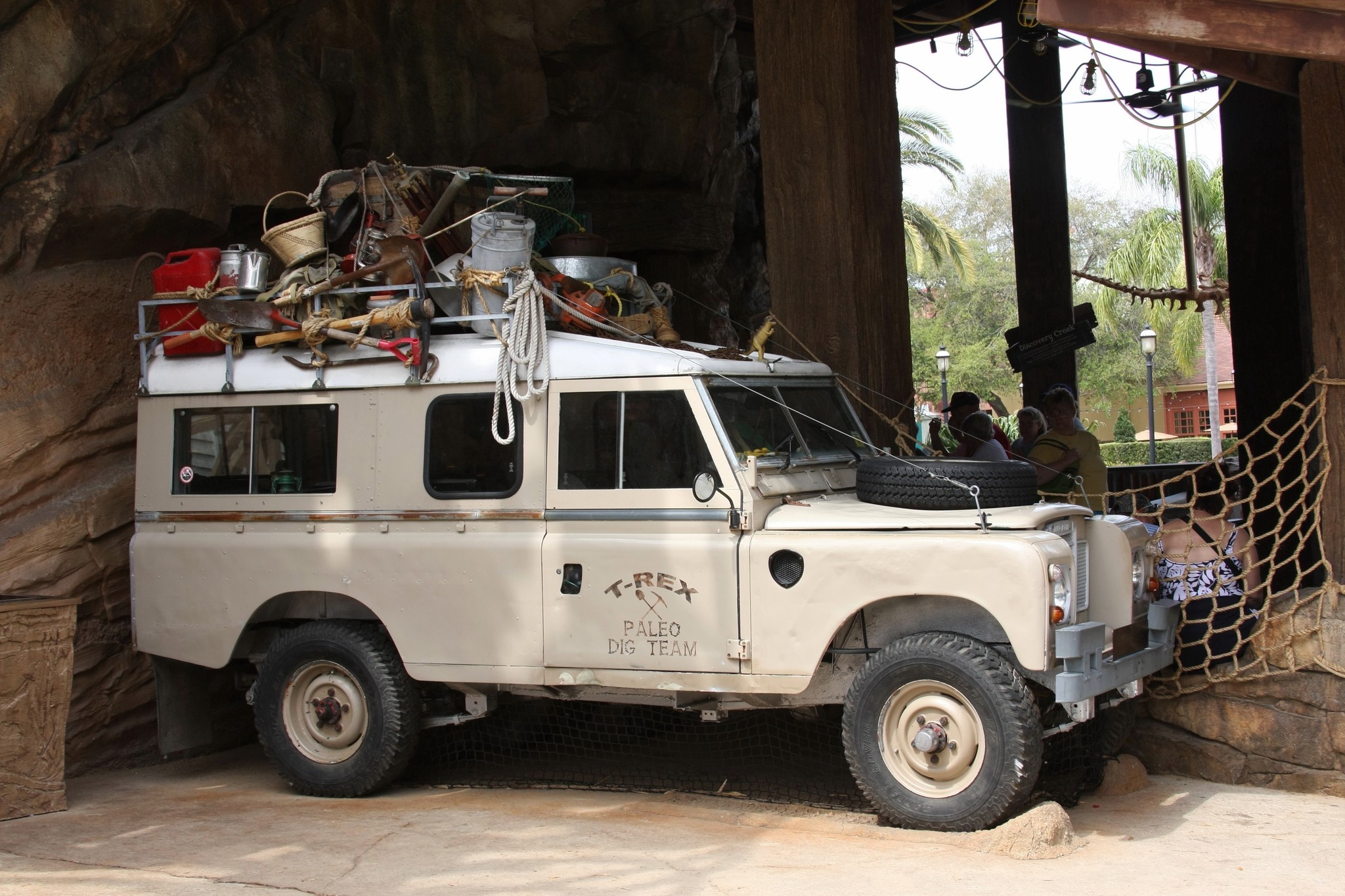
[
  {"x1": 327, "y1": 174, "x2": 364, "y2": 246},
  {"x1": 552, "y1": 229, "x2": 608, "y2": 257}
]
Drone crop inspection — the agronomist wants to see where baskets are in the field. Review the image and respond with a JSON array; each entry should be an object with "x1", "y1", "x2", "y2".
[{"x1": 260, "y1": 191, "x2": 328, "y2": 268}]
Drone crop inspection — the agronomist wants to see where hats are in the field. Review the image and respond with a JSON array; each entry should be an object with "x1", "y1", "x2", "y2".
[{"x1": 941, "y1": 392, "x2": 980, "y2": 413}]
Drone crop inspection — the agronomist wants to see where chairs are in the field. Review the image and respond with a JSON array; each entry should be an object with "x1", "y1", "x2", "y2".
[{"x1": 624, "y1": 422, "x2": 676, "y2": 488}]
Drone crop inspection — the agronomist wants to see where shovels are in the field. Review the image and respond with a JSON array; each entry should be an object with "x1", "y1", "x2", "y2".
[{"x1": 196, "y1": 298, "x2": 422, "y2": 366}]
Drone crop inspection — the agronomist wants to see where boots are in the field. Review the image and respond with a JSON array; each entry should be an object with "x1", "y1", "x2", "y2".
[
  {"x1": 603, "y1": 313, "x2": 651, "y2": 335},
  {"x1": 651, "y1": 307, "x2": 680, "y2": 342}
]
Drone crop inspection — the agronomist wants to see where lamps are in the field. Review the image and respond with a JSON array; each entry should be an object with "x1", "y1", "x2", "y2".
[{"x1": 271, "y1": 469, "x2": 303, "y2": 494}]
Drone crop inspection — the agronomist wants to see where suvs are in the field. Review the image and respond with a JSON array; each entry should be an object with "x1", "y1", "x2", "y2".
[{"x1": 129, "y1": 275, "x2": 1185, "y2": 832}]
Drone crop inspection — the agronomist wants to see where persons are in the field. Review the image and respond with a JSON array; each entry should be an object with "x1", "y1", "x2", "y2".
[
  {"x1": 1152, "y1": 464, "x2": 1263, "y2": 676},
  {"x1": 916, "y1": 384, "x2": 1110, "y2": 515},
  {"x1": 1110, "y1": 493, "x2": 1159, "y2": 536}
]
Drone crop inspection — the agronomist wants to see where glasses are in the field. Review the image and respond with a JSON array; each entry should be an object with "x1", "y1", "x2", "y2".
[
  {"x1": 1017, "y1": 421, "x2": 1037, "y2": 427},
  {"x1": 962, "y1": 432, "x2": 969, "y2": 436}
]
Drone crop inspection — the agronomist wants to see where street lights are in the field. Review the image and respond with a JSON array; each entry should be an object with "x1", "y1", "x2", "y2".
[
  {"x1": 935, "y1": 342, "x2": 951, "y2": 424},
  {"x1": 1139, "y1": 320, "x2": 1158, "y2": 465}
]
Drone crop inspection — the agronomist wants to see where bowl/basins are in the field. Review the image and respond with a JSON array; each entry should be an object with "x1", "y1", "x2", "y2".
[{"x1": 426, "y1": 253, "x2": 473, "y2": 327}]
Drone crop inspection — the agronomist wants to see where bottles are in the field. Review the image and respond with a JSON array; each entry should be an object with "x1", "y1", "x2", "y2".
[{"x1": 150, "y1": 247, "x2": 226, "y2": 357}]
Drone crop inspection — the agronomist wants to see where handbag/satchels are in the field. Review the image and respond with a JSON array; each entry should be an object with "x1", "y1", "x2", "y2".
[{"x1": 1031, "y1": 438, "x2": 1078, "y2": 498}]
